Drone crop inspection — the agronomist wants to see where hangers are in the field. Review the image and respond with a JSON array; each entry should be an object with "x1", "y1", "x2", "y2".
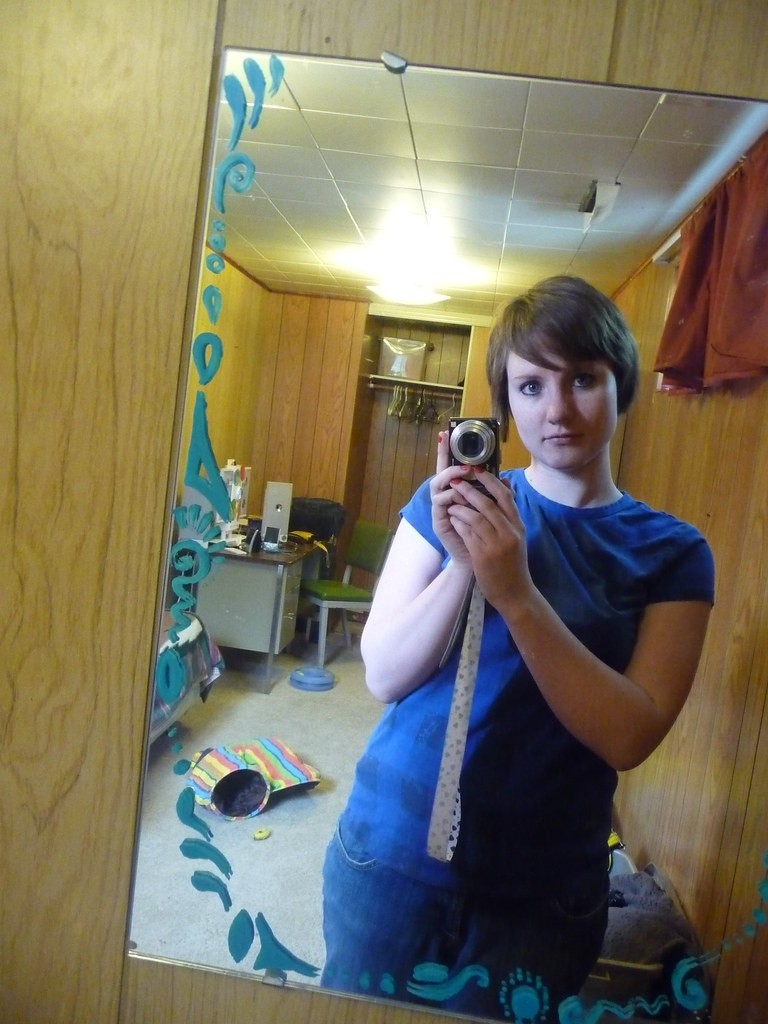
[{"x1": 386, "y1": 383, "x2": 459, "y2": 425}]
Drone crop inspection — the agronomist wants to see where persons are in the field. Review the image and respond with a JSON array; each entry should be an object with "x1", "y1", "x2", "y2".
[{"x1": 320, "y1": 275, "x2": 716, "y2": 1024}]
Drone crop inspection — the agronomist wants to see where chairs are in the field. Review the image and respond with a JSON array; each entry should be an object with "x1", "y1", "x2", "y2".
[{"x1": 285, "y1": 518, "x2": 396, "y2": 667}]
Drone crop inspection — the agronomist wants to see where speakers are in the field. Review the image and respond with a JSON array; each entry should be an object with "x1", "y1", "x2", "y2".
[
  {"x1": 243, "y1": 526, "x2": 262, "y2": 553},
  {"x1": 262, "y1": 525, "x2": 281, "y2": 553}
]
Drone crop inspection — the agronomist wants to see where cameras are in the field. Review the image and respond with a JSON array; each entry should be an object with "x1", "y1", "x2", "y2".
[{"x1": 443, "y1": 414, "x2": 499, "y2": 504}]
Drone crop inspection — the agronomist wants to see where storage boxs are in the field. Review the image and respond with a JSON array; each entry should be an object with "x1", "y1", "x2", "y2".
[{"x1": 377, "y1": 336, "x2": 427, "y2": 381}]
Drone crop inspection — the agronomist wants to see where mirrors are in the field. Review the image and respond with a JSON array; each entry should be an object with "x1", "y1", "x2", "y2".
[{"x1": 119, "y1": 47, "x2": 768, "y2": 1024}]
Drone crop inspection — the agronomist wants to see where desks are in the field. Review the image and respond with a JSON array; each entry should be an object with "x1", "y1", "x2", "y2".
[{"x1": 188, "y1": 540, "x2": 326, "y2": 694}]
